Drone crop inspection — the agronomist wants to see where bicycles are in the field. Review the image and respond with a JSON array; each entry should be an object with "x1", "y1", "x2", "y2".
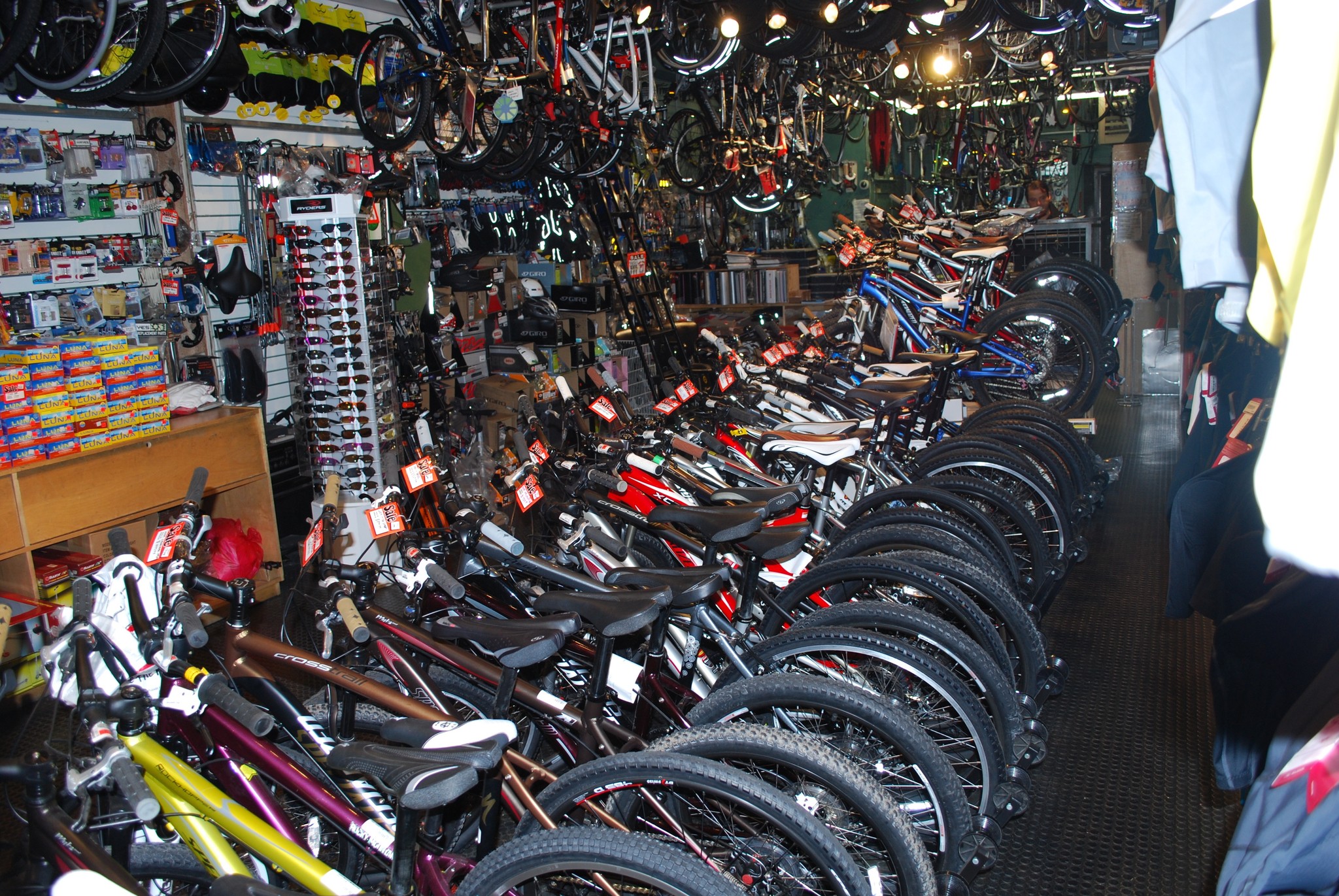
[
  {"x1": 0, "y1": 183, "x2": 1134, "y2": 896},
  {"x1": 345, "y1": 0, "x2": 1160, "y2": 215},
  {"x1": 0, "y1": 0, "x2": 227, "y2": 108}
]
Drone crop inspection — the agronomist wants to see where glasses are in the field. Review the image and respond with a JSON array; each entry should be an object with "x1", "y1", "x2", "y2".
[
  {"x1": 288, "y1": 268, "x2": 323, "y2": 280},
  {"x1": 290, "y1": 282, "x2": 332, "y2": 294},
  {"x1": 326, "y1": 279, "x2": 357, "y2": 294},
  {"x1": 327, "y1": 294, "x2": 358, "y2": 307},
  {"x1": 288, "y1": 347, "x2": 379, "y2": 505},
  {"x1": 328, "y1": 321, "x2": 361, "y2": 335},
  {"x1": 327, "y1": 307, "x2": 357, "y2": 319},
  {"x1": 282, "y1": 226, "x2": 318, "y2": 239},
  {"x1": 287, "y1": 239, "x2": 326, "y2": 252},
  {"x1": 295, "y1": 323, "x2": 329, "y2": 340},
  {"x1": 324, "y1": 265, "x2": 355, "y2": 279},
  {"x1": 321, "y1": 251, "x2": 352, "y2": 266},
  {"x1": 293, "y1": 309, "x2": 331, "y2": 319},
  {"x1": 295, "y1": 336, "x2": 330, "y2": 346},
  {"x1": 288, "y1": 255, "x2": 321, "y2": 267},
  {"x1": 321, "y1": 223, "x2": 353, "y2": 239},
  {"x1": 328, "y1": 334, "x2": 362, "y2": 348},
  {"x1": 320, "y1": 237, "x2": 352, "y2": 252},
  {"x1": 290, "y1": 295, "x2": 325, "y2": 310}
]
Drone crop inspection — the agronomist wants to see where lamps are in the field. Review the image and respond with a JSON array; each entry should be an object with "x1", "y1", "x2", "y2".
[
  {"x1": 818, "y1": 0, "x2": 839, "y2": 24},
  {"x1": 944, "y1": 0, "x2": 958, "y2": 8},
  {"x1": 677, "y1": 3, "x2": 702, "y2": 37},
  {"x1": 1038, "y1": 38, "x2": 1073, "y2": 95},
  {"x1": 871, "y1": 0, "x2": 892, "y2": 12},
  {"x1": 713, "y1": 0, "x2": 741, "y2": 39},
  {"x1": 629, "y1": 0, "x2": 654, "y2": 25},
  {"x1": 803, "y1": 59, "x2": 874, "y2": 110},
  {"x1": 893, "y1": 51, "x2": 910, "y2": 79},
  {"x1": 766, "y1": 0, "x2": 788, "y2": 30},
  {"x1": 911, "y1": 78, "x2": 1028, "y2": 110}
]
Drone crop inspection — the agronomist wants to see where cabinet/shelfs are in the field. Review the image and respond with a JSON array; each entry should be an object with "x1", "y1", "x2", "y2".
[
  {"x1": 667, "y1": 264, "x2": 835, "y2": 326},
  {"x1": 0, "y1": 406, "x2": 285, "y2": 717}
]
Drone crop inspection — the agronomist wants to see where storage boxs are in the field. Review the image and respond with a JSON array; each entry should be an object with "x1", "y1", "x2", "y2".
[
  {"x1": 675, "y1": 308, "x2": 752, "y2": 335},
  {"x1": 0, "y1": 512, "x2": 161, "y2": 703},
  {"x1": 0, "y1": 334, "x2": 170, "y2": 469},
  {"x1": 387, "y1": 253, "x2": 628, "y2": 455}
]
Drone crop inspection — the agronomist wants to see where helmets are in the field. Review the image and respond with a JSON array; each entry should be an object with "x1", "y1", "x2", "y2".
[
  {"x1": 521, "y1": 278, "x2": 545, "y2": 297},
  {"x1": 516, "y1": 345, "x2": 539, "y2": 365},
  {"x1": 523, "y1": 296, "x2": 561, "y2": 320}
]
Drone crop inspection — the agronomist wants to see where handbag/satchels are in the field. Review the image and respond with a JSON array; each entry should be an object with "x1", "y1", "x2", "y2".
[{"x1": 206, "y1": 517, "x2": 264, "y2": 584}]
